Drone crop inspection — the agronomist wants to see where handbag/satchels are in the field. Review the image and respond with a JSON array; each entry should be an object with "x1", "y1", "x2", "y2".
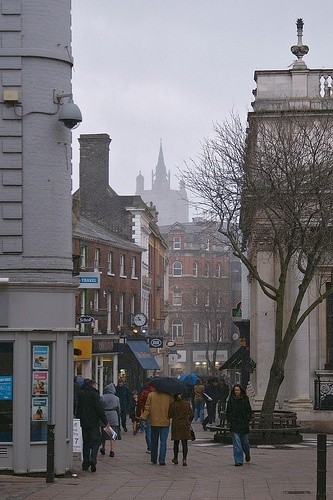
[{"x1": 190, "y1": 426, "x2": 196, "y2": 442}]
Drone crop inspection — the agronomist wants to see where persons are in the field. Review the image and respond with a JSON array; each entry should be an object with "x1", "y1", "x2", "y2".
[
  {"x1": 36, "y1": 382, "x2": 44, "y2": 390},
  {"x1": 116, "y1": 379, "x2": 155, "y2": 453},
  {"x1": 144, "y1": 389, "x2": 174, "y2": 465},
  {"x1": 184, "y1": 377, "x2": 229, "y2": 430},
  {"x1": 35, "y1": 356, "x2": 44, "y2": 365},
  {"x1": 36, "y1": 407, "x2": 43, "y2": 418},
  {"x1": 73, "y1": 376, "x2": 110, "y2": 472},
  {"x1": 99, "y1": 383, "x2": 121, "y2": 456},
  {"x1": 168, "y1": 392, "x2": 195, "y2": 466},
  {"x1": 226, "y1": 384, "x2": 252, "y2": 466}
]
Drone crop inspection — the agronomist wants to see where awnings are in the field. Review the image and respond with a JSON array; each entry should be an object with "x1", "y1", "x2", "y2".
[
  {"x1": 124, "y1": 340, "x2": 160, "y2": 370},
  {"x1": 219, "y1": 346, "x2": 246, "y2": 370}
]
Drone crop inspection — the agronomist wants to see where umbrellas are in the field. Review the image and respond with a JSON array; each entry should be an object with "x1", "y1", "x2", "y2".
[
  {"x1": 154, "y1": 376, "x2": 187, "y2": 395},
  {"x1": 179, "y1": 374, "x2": 205, "y2": 385}
]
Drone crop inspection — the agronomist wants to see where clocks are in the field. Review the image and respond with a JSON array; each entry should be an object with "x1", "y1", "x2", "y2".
[{"x1": 134, "y1": 314, "x2": 147, "y2": 327}]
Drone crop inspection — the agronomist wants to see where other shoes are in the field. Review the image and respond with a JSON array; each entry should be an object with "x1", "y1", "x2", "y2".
[
  {"x1": 202, "y1": 422, "x2": 207, "y2": 431},
  {"x1": 91, "y1": 460, "x2": 97, "y2": 472},
  {"x1": 100, "y1": 448, "x2": 105, "y2": 455},
  {"x1": 110, "y1": 451, "x2": 114, "y2": 457},
  {"x1": 235, "y1": 463, "x2": 243, "y2": 466},
  {"x1": 172, "y1": 458, "x2": 178, "y2": 464},
  {"x1": 146, "y1": 450, "x2": 151, "y2": 453},
  {"x1": 133, "y1": 431, "x2": 136, "y2": 436},
  {"x1": 182, "y1": 461, "x2": 187, "y2": 466},
  {"x1": 245, "y1": 451, "x2": 250, "y2": 461},
  {"x1": 159, "y1": 463, "x2": 166, "y2": 465}
]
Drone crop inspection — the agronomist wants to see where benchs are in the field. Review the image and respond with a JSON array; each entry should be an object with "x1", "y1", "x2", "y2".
[{"x1": 206, "y1": 423, "x2": 303, "y2": 433}]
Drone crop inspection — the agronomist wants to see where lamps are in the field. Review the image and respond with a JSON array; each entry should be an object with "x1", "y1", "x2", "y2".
[
  {"x1": 156, "y1": 309, "x2": 169, "y2": 320},
  {"x1": 239, "y1": 336, "x2": 247, "y2": 347}
]
